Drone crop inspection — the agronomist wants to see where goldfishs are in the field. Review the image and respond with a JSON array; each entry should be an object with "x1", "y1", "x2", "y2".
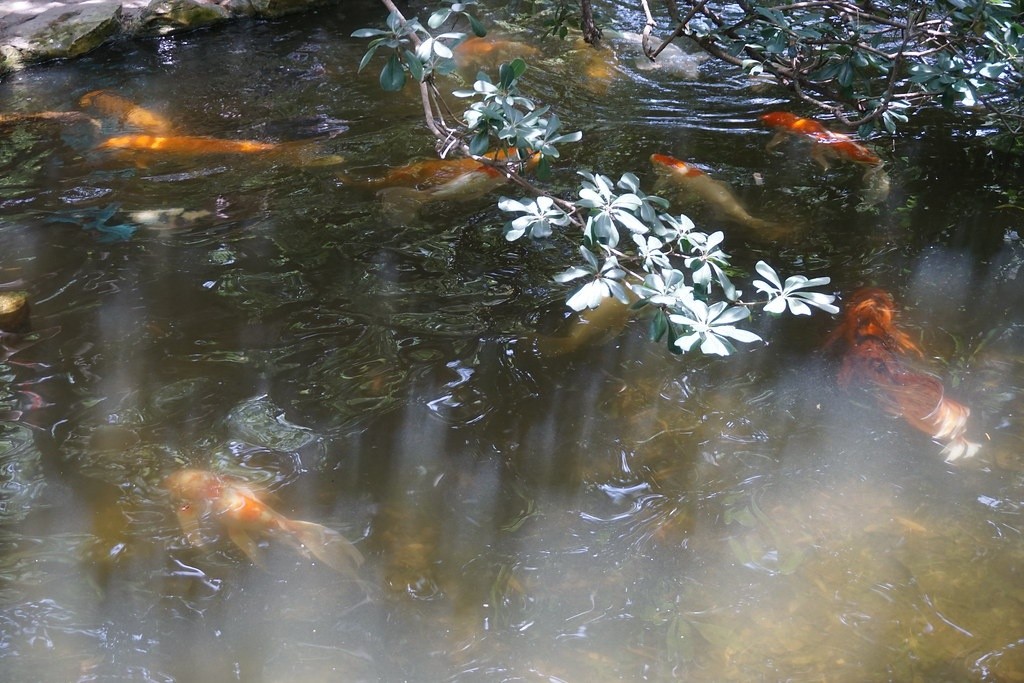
[
  {"x1": 0, "y1": 87, "x2": 351, "y2": 167},
  {"x1": 329, "y1": 34, "x2": 973, "y2": 443},
  {"x1": 157, "y1": 467, "x2": 368, "y2": 577}
]
